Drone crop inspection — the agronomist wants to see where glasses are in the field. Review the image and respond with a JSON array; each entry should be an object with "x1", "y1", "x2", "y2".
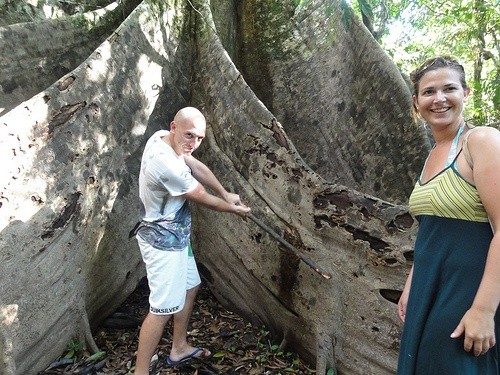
[{"x1": 415, "y1": 56, "x2": 465, "y2": 95}]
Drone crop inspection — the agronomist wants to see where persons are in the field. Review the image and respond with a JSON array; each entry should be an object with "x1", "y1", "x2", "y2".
[
  {"x1": 135, "y1": 107, "x2": 251, "y2": 375},
  {"x1": 397, "y1": 57, "x2": 500, "y2": 375}
]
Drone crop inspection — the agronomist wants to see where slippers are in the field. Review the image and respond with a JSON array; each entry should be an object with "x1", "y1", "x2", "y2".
[{"x1": 167, "y1": 347, "x2": 209, "y2": 364}]
